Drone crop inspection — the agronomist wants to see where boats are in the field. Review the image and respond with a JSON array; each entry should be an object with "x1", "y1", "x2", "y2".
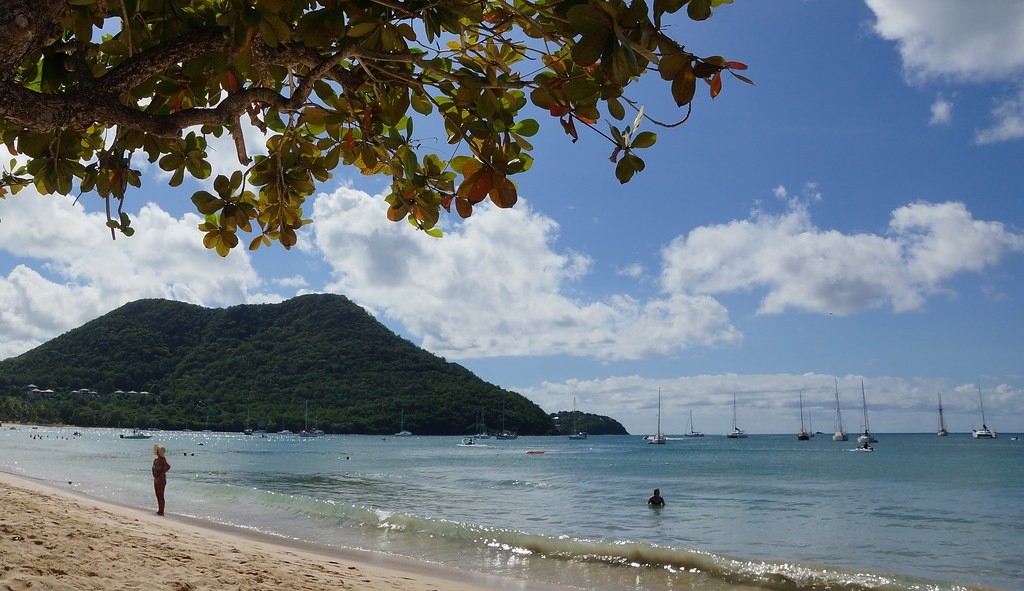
[
  {"x1": 462, "y1": 437, "x2": 475, "y2": 446},
  {"x1": 856, "y1": 445, "x2": 873, "y2": 452},
  {"x1": 119, "y1": 428, "x2": 152, "y2": 440},
  {"x1": 1011, "y1": 437, "x2": 1020, "y2": 441}
]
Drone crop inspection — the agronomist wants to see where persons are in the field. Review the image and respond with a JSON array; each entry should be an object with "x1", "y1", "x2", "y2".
[
  {"x1": 648, "y1": 488, "x2": 665, "y2": 506},
  {"x1": 152, "y1": 444, "x2": 171, "y2": 516},
  {"x1": 863, "y1": 442, "x2": 871, "y2": 449}
]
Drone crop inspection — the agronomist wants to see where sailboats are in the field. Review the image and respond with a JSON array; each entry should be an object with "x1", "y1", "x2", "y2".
[
  {"x1": 567, "y1": 397, "x2": 588, "y2": 439},
  {"x1": 276, "y1": 412, "x2": 292, "y2": 434},
  {"x1": 243, "y1": 409, "x2": 253, "y2": 435},
  {"x1": 203, "y1": 409, "x2": 211, "y2": 431},
  {"x1": 685, "y1": 410, "x2": 704, "y2": 437},
  {"x1": 858, "y1": 379, "x2": 875, "y2": 443},
  {"x1": 299, "y1": 399, "x2": 324, "y2": 437},
  {"x1": 495, "y1": 399, "x2": 517, "y2": 439},
  {"x1": 798, "y1": 390, "x2": 810, "y2": 440},
  {"x1": 184, "y1": 414, "x2": 191, "y2": 432},
  {"x1": 971, "y1": 382, "x2": 997, "y2": 439},
  {"x1": 473, "y1": 407, "x2": 489, "y2": 438},
  {"x1": 832, "y1": 376, "x2": 849, "y2": 441},
  {"x1": 394, "y1": 409, "x2": 412, "y2": 437},
  {"x1": 937, "y1": 392, "x2": 947, "y2": 436},
  {"x1": 646, "y1": 387, "x2": 667, "y2": 444},
  {"x1": 724, "y1": 391, "x2": 748, "y2": 438}
]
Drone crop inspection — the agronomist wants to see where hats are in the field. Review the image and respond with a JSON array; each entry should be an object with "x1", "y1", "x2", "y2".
[{"x1": 153, "y1": 444, "x2": 167, "y2": 458}]
